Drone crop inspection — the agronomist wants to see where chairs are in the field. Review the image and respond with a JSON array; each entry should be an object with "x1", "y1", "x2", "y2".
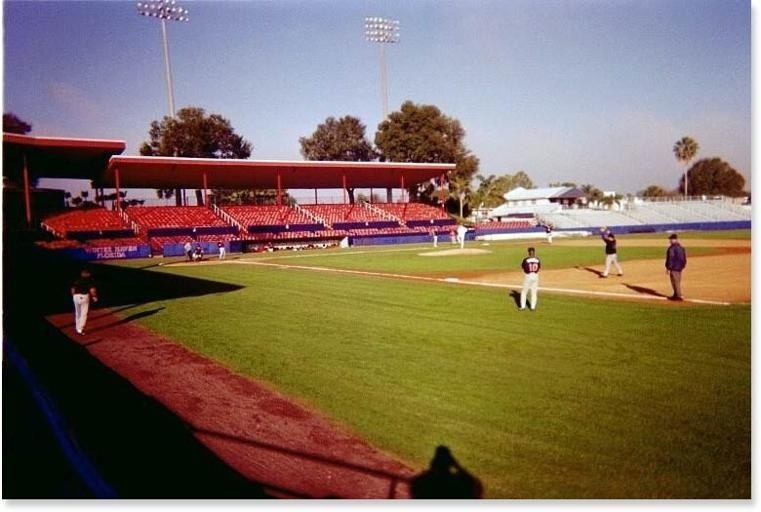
[
  {"x1": 40, "y1": 202, "x2": 458, "y2": 248},
  {"x1": 475, "y1": 220, "x2": 532, "y2": 232}
]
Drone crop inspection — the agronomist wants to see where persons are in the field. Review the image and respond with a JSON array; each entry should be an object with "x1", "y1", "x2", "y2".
[
  {"x1": 72, "y1": 269, "x2": 98, "y2": 335},
  {"x1": 263, "y1": 242, "x2": 273, "y2": 253},
  {"x1": 665, "y1": 234, "x2": 686, "y2": 300},
  {"x1": 184, "y1": 240, "x2": 192, "y2": 262},
  {"x1": 217, "y1": 240, "x2": 225, "y2": 260},
  {"x1": 541, "y1": 223, "x2": 554, "y2": 243},
  {"x1": 433, "y1": 228, "x2": 437, "y2": 247},
  {"x1": 193, "y1": 244, "x2": 203, "y2": 261},
  {"x1": 457, "y1": 223, "x2": 467, "y2": 248},
  {"x1": 600, "y1": 228, "x2": 623, "y2": 278},
  {"x1": 449, "y1": 230, "x2": 456, "y2": 243},
  {"x1": 519, "y1": 248, "x2": 541, "y2": 311}
]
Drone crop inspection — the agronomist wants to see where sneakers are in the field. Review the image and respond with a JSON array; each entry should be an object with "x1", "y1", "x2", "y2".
[
  {"x1": 669, "y1": 294, "x2": 683, "y2": 302},
  {"x1": 600, "y1": 275, "x2": 608, "y2": 279},
  {"x1": 617, "y1": 273, "x2": 624, "y2": 276}
]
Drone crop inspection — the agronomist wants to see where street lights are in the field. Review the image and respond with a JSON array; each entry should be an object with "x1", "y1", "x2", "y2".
[
  {"x1": 136, "y1": 0, "x2": 190, "y2": 156},
  {"x1": 362, "y1": 16, "x2": 402, "y2": 202}
]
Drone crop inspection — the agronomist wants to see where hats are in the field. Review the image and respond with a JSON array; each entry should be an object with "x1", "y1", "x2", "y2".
[{"x1": 669, "y1": 233, "x2": 677, "y2": 240}]
